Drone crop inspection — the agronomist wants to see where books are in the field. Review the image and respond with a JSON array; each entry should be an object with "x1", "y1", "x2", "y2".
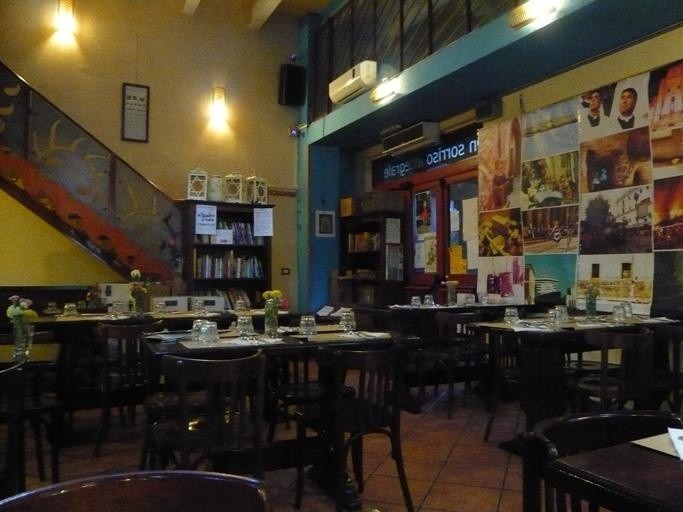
[
  {"x1": 216, "y1": 222, "x2": 254, "y2": 245},
  {"x1": 208, "y1": 288, "x2": 251, "y2": 310},
  {"x1": 193, "y1": 248, "x2": 265, "y2": 279},
  {"x1": 348, "y1": 232, "x2": 379, "y2": 253}
]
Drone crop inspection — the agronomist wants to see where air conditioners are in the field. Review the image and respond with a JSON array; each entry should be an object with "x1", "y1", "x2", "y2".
[
  {"x1": 381, "y1": 122, "x2": 439, "y2": 158},
  {"x1": 329, "y1": 60, "x2": 377, "y2": 105}
]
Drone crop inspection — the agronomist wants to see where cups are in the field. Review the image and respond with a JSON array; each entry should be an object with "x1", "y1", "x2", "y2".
[
  {"x1": 192, "y1": 319, "x2": 208, "y2": 339},
  {"x1": 465, "y1": 296, "x2": 473, "y2": 306},
  {"x1": 612, "y1": 306, "x2": 624, "y2": 321},
  {"x1": 236, "y1": 316, "x2": 254, "y2": 334},
  {"x1": 549, "y1": 309, "x2": 561, "y2": 326},
  {"x1": 233, "y1": 301, "x2": 246, "y2": 313},
  {"x1": 155, "y1": 303, "x2": 164, "y2": 314},
  {"x1": 113, "y1": 303, "x2": 123, "y2": 317},
  {"x1": 504, "y1": 308, "x2": 518, "y2": 323},
  {"x1": 340, "y1": 311, "x2": 356, "y2": 331},
  {"x1": 555, "y1": 305, "x2": 568, "y2": 320},
  {"x1": 200, "y1": 322, "x2": 218, "y2": 343},
  {"x1": 193, "y1": 301, "x2": 205, "y2": 314},
  {"x1": 300, "y1": 317, "x2": 316, "y2": 335},
  {"x1": 482, "y1": 296, "x2": 488, "y2": 304},
  {"x1": 47, "y1": 302, "x2": 56, "y2": 310},
  {"x1": 424, "y1": 294, "x2": 433, "y2": 304},
  {"x1": 64, "y1": 303, "x2": 76, "y2": 314},
  {"x1": 411, "y1": 297, "x2": 421, "y2": 307},
  {"x1": 621, "y1": 303, "x2": 632, "y2": 317}
]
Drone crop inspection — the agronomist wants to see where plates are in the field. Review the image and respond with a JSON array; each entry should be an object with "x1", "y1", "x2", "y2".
[{"x1": 43, "y1": 310, "x2": 61, "y2": 314}]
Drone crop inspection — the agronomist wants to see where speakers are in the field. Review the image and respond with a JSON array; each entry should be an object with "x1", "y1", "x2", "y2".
[{"x1": 279, "y1": 64, "x2": 305, "y2": 106}]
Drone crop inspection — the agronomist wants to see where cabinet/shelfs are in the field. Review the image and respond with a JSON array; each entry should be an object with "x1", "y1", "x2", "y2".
[
  {"x1": 185, "y1": 197, "x2": 273, "y2": 310},
  {"x1": 340, "y1": 212, "x2": 408, "y2": 305}
]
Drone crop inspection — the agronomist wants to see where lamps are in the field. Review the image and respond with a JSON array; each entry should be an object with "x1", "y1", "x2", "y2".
[
  {"x1": 370, "y1": 76, "x2": 398, "y2": 103},
  {"x1": 508, "y1": 2, "x2": 554, "y2": 30},
  {"x1": 212, "y1": 85, "x2": 224, "y2": 115},
  {"x1": 55, "y1": 1, "x2": 75, "y2": 30}
]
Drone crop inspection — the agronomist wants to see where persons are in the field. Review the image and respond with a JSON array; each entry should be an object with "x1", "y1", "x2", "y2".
[
  {"x1": 579, "y1": 88, "x2": 612, "y2": 140},
  {"x1": 612, "y1": 88, "x2": 646, "y2": 134},
  {"x1": 493, "y1": 160, "x2": 508, "y2": 209}
]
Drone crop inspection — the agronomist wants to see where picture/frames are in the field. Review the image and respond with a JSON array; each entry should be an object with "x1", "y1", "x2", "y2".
[
  {"x1": 315, "y1": 209, "x2": 336, "y2": 238},
  {"x1": 122, "y1": 82, "x2": 150, "y2": 142}
]
